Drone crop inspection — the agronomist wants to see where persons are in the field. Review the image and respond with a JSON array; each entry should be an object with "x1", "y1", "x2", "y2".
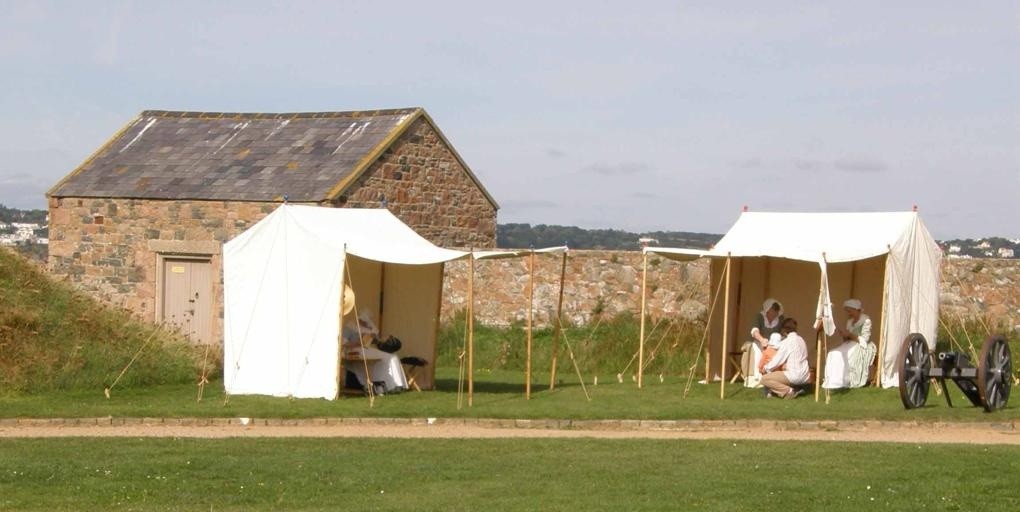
[
  {"x1": 758, "y1": 332, "x2": 786, "y2": 398},
  {"x1": 760, "y1": 317, "x2": 811, "y2": 401},
  {"x1": 342, "y1": 306, "x2": 409, "y2": 394},
  {"x1": 820, "y1": 298, "x2": 872, "y2": 390},
  {"x1": 741, "y1": 298, "x2": 785, "y2": 389}
]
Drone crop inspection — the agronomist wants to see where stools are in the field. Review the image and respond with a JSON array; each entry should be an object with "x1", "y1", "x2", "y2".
[
  {"x1": 399, "y1": 356, "x2": 429, "y2": 393},
  {"x1": 728, "y1": 351, "x2": 746, "y2": 385}
]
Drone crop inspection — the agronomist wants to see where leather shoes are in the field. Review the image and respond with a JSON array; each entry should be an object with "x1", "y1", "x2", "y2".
[{"x1": 784, "y1": 388, "x2": 794, "y2": 401}]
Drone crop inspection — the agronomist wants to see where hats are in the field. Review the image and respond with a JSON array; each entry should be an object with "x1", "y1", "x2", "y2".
[
  {"x1": 768, "y1": 333, "x2": 781, "y2": 346},
  {"x1": 843, "y1": 299, "x2": 865, "y2": 313}
]
